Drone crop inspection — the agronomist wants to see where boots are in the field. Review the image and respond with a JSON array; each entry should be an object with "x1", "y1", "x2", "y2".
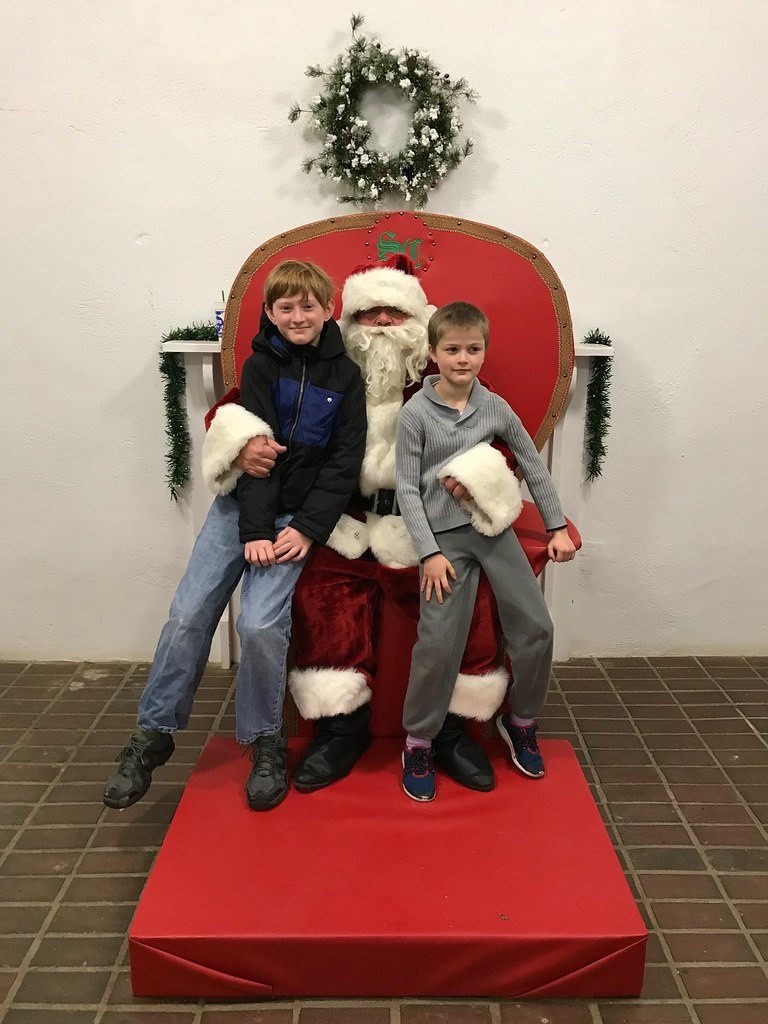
[
  {"x1": 431, "y1": 711, "x2": 494, "y2": 792},
  {"x1": 292, "y1": 704, "x2": 372, "y2": 792}
]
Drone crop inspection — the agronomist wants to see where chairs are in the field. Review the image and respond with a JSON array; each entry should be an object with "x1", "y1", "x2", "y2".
[{"x1": 221, "y1": 211, "x2": 577, "y2": 730}]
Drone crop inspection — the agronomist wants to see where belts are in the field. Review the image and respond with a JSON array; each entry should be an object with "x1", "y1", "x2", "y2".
[{"x1": 349, "y1": 488, "x2": 401, "y2": 516}]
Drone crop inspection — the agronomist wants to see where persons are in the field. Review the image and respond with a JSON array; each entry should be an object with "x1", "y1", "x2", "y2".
[
  {"x1": 196, "y1": 253, "x2": 527, "y2": 794},
  {"x1": 101, "y1": 259, "x2": 369, "y2": 813},
  {"x1": 395, "y1": 301, "x2": 578, "y2": 803}
]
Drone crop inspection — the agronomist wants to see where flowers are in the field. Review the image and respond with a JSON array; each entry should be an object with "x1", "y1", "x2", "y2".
[{"x1": 288, "y1": 12, "x2": 482, "y2": 214}]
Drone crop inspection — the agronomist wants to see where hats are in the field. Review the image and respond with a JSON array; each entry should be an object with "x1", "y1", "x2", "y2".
[{"x1": 340, "y1": 253, "x2": 428, "y2": 322}]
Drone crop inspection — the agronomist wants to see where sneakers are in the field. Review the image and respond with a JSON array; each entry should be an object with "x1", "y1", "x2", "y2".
[
  {"x1": 401, "y1": 745, "x2": 436, "y2": 802},
  {"x1": 103, "y1": 728, "x2": 175, "y2": 809},
  {"x1": 496, "y1": 709, "x2": 545, "y2": 777},
  {"x1": 242, "y1": 728, "x2": 289, "y2": 811}
]
